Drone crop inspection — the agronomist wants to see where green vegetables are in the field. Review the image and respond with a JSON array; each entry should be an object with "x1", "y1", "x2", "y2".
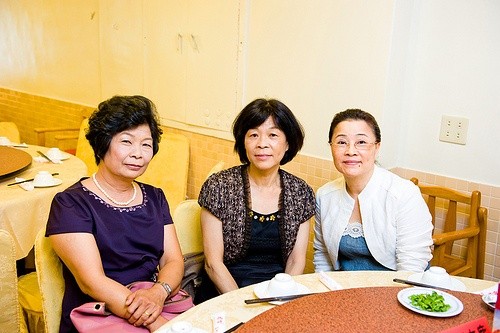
[{"x1": 410, "y1": 291, "x2": 450, "y2": 312}]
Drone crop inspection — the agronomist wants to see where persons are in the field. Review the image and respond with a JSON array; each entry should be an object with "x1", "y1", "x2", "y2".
[
  {"x1": 192, "y1": 98, "x2": 317, "y2": 307},
  {"x1": 44, "y1": 95, "x2": 185, "y2": 333},
  {"x1": 312, "y1": 109, "x2": 435, "y2": 273}
]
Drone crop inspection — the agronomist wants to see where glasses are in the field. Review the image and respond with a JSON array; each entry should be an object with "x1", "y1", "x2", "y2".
[{"x1": 328, "y1": 139, "x2": 379, "y2": 151}]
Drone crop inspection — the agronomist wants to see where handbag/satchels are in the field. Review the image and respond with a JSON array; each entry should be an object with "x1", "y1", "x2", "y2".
[{"x1": 69, "y1": 281, "x2": 196, "y2": 333}]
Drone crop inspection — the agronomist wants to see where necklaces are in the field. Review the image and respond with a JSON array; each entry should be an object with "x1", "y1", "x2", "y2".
[{"x1": 92, "y1": 172, "x2": 138, "y2": 206}]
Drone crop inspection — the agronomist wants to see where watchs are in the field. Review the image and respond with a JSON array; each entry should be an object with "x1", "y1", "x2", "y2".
[{"x1": 152, "y1": 281, "x2": 172, "y2": 299}]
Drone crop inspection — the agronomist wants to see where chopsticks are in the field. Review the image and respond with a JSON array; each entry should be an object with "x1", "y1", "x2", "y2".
[
  {"x1": 393, "y1": 278, "x2": 436, "y2": 289},
  {"x1": 36, "y1": 150, "x2": 55, "y2": 165},
  {"x1": 7, "y1": 173, "x2": 59, "y2": 186},
  {"x1": 245, "y1": 293, "x2": 311, "y2": 305}
]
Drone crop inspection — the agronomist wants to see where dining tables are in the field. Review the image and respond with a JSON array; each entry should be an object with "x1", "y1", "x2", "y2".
[
  {"x1": 148, "y1": 271, "x2": 500, "y2": 333},
  {"x1": 0, "y1": 140, "x2": 87, "y2": 262}
]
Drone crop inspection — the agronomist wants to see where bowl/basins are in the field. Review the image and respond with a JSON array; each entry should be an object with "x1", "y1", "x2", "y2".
[
  {"x1": 267, "y1": 273, "x2": 296, "y2": 296},
  {"x1": 35, "y1": 170, "x2": 55, "y2": 184},
  {"x1": 47, "y1": 147, "x2": 62, "y2": 160},
  {"x1": 422, "y1": 266, "x2": 452, "y2": 284},
  {"x1": 0, "y1": 135, "x2": 11, "y2": 146}
]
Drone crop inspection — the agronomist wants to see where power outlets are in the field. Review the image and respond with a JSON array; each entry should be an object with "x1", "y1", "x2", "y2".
[{"x1": 439, "y1": 116, "x2": 469, "y2": 144}]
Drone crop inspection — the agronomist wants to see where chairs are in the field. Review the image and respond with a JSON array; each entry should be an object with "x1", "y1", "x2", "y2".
[
  {"x1": 0, "y1": 120, "x2": 203, "y2": 333},
  {"x1": 407, "y1": 180, "x2": 488, "y2": 279}
]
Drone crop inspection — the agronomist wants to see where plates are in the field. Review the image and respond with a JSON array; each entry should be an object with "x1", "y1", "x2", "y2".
[
  {"x1": 398, "y1": 287, "x2": 463, "y2": 319},
  {"x1": 60, "y1": 154, "x2": 69, "y2": 161},
  {"x1": 253, "y1": 281, "x2": 308, "y2": 306},
  {"x1": 32, "y1": 179, "x2": 63, "y2": 188},
  {"x1": 481, "y1": 294, "x2": 497, "y2": 310}
]
